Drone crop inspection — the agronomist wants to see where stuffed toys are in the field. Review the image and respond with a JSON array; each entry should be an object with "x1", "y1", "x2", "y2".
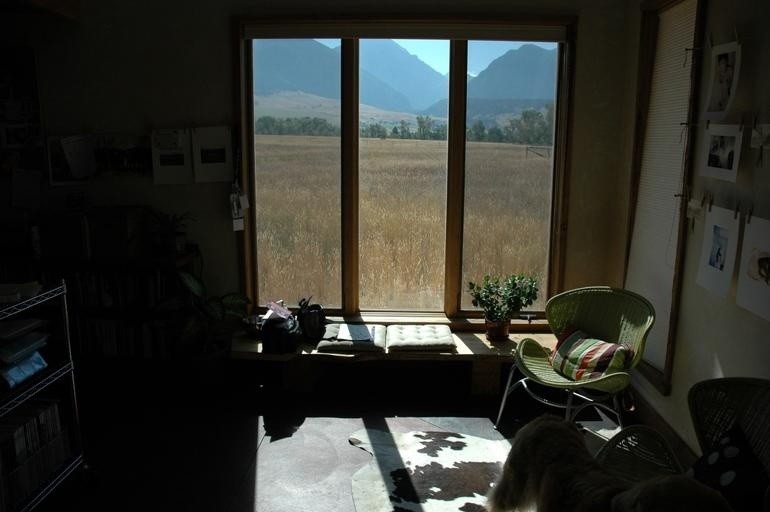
[{"x1": 485, "y1": 413, "x2": 728, "y2": 511}]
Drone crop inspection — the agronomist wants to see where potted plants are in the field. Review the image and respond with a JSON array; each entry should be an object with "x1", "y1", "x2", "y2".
[{"x1": 463, "y1": 272, "x2": 542, "y2": 342}]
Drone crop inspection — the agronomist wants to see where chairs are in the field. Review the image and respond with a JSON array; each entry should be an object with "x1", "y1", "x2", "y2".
[
  {"x1": 491, "y1": 286, "x2": 658, "y2": 433},
  {"x1": 588, "y1": 374, "x2": 770, "y2": 512}
]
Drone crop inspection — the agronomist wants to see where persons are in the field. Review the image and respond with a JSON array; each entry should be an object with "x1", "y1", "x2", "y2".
[
  {"x1": 713, "y1": 245, "x2": 724, "y2": 268},
  {"x1": 716, "y1": 52, "x2": 733, "y2": 103}
]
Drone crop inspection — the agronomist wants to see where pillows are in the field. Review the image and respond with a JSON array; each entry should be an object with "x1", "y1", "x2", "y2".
[
  {"x1": 547, "y1": 324, "x2": 635, "y2": 395},
  {"x1": 692, "y1": 424, "x2": 766, "y2": 509}
]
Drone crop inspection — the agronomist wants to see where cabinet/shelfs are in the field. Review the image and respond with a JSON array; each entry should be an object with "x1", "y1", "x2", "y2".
[
  {"x1": 0, "y1": 277, "x2": 88, "y2": 512},
  {"x1": 4, "y1": 207, "x2": 207, "y2": 368}
]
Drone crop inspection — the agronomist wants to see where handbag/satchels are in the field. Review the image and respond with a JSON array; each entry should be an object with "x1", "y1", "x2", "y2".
[{"x1": 261, "y1": 310, "x2": 300, "y2": 355}]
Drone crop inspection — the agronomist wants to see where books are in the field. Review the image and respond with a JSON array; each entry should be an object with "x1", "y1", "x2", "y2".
[{"x1": 1, "y1": 401, "x2": 76, "y2": 508}]
